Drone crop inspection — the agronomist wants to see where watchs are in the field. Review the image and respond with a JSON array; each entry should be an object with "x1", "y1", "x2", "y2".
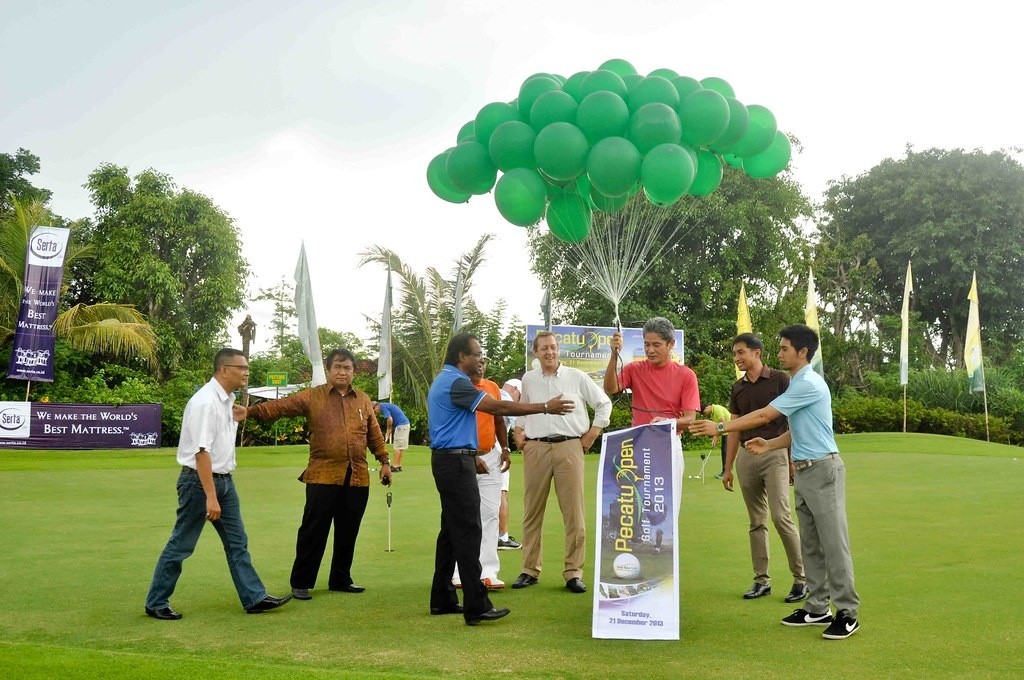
[{"x1": 717, "y1": 421, "x2": 724, "y2": 433}]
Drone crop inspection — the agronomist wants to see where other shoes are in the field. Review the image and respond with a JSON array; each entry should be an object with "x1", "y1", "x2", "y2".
[
  {"x1": 715, "y1": 470, "x2": 725, "y2": 479},
  {"x1": 390, "y1": 466, "x2": 401, "y2": 472}
]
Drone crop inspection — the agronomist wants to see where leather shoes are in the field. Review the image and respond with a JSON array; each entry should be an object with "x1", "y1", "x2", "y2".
[
  {"x1": 785, "y1": 581, "x2": 809, "y2": 602},
  {"x1": 743, "y1": 582, "x2": 770, "y2": 598},
  {"x1": 247, "y1": 594, "x2": 294, "y2": 614},
  {"x1": 329, "y1": 582, "x2": 365, "y2": 593},
  {"x1": 566, "y1": 577, "x2": 586, "y2": 591},
  {"x1": 466, "y1": 606, "x2": 510, "y2": 625},
  {"x1": 292, "y1": 586, "x2": 313, "y2": 600},
  {"x1": 512, "y1": 573, "x2": 538, "y2": 588},
  {"x1": 430, "y1": 605, "x2": 466, "y2": 614},
  {"x1": 145, "y1": 605, "x2": 182, "y2": 620}
]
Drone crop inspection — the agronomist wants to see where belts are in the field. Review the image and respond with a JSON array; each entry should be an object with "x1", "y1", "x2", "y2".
[
  {"x1": 793, "y1": 452, "x2": 839, "y2": 471},
  {"x1": 432, "y1": 448, "x2": 478, "y2": 456},
  {"x1": 527, "y1": 436, "x2": 579, "y2": 443}
]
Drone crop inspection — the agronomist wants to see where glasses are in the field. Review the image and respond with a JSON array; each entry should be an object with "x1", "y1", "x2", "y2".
[{"x1": 218, "y1": 364, "x2": 250, "y2": 373}]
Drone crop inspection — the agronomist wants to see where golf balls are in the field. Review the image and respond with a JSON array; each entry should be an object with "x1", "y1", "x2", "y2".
[{"x1": 613, "y1": 553, "x2": 642, "y2": 580}]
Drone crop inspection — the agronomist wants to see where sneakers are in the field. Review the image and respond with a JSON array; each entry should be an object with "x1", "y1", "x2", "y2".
[
  {"x1": 782, "y1": 602, "x2": 833, "y2": 627},
  {"x1": 482, "y1": 577, "x2": 506, "y2": 589},
  {"x1": 498, "y1": 536, "x2": 522, "y2": 550},
  {"x1": 823, "y1": 611, "x2": 860, "y2": 640}
]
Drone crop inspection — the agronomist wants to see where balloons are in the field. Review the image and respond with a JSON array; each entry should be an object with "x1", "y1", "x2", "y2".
[{"x1": 427, "y1": 58, "x2": 791, "y2": 246}]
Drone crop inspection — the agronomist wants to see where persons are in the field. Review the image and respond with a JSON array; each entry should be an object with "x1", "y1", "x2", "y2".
[
  {"x1": 145, "y1": 348, "x2": 292, "y2": 621},
  {"x1": 371, "y1": 401, "x2": 410, "y2": 472},
  {"x1": 722, "y1": 333, "x2": 809, "y2": 603},
  {"x1": 688, "y1": 326, "x2": 861, "y2": 639},
  {"x1": 603, "y1": 316, "x2": 701, "y2": 518},
  {"x1": 496, "y1": 378, "x2": 522, "y2": 550},
  {"x1": 698, "y1": 403, "x2": 731, "y2": 479},
  {"x1": 511, "y1": 331, "x2": 613, "y2": 594},
  {"x1": 427, "y1": 334, "x2": 575, "y2": 626},
  {"x1": 232, "y1": 348, "x2": 392, "y2": 601}
]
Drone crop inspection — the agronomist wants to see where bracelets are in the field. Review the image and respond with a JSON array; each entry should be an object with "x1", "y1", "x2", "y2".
[
  {"x1": 502, "y1": 447, "x2": 511, "y2": 454},
  {"x1": 544, "y1": 402, "x2": 547, "y2": 414}
]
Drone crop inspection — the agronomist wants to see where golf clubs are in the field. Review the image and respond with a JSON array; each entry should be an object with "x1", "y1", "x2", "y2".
[
  {"x1": 695, "y1": 447, "x2": 714, "y2": 479},
  {"x1": 371, "y1": 433, "x2": 388, "y2": 472}
]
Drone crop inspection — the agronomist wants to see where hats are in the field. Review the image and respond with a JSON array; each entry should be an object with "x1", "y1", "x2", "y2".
[
  {"x1": 371, "y1": 400, "x2": 376, "y2": 409},
  {"x1": 505, "y1": 379, "x2": 523, "y2": 393}
]
